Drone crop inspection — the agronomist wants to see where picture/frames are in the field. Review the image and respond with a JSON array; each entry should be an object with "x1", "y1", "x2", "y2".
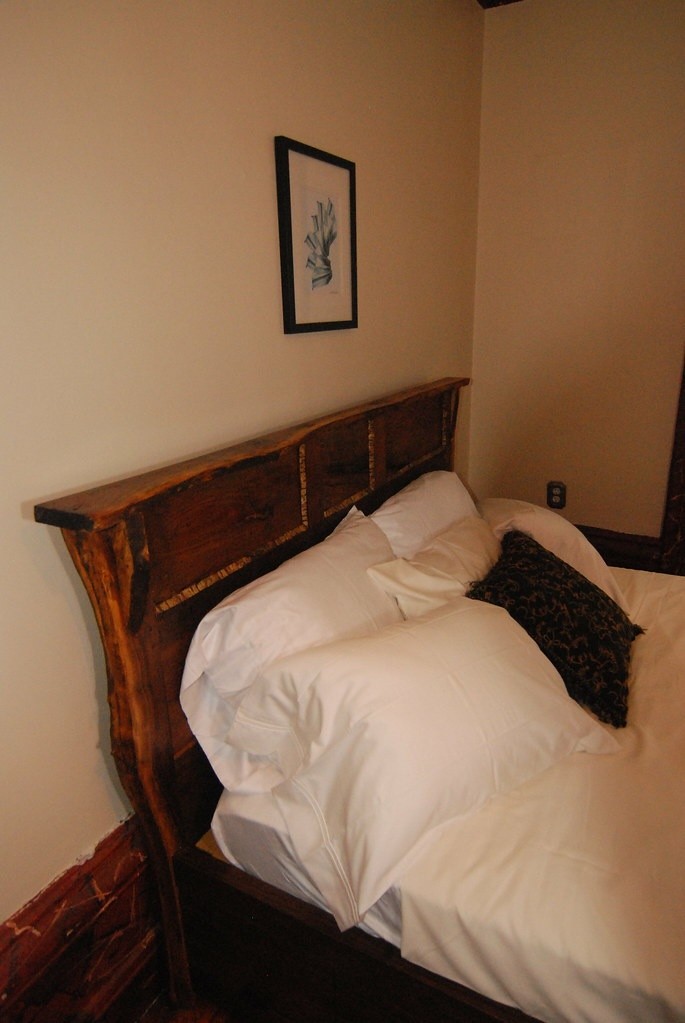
[{"x1": 274, "y1": 134, "x2": 358, "y2": 335}]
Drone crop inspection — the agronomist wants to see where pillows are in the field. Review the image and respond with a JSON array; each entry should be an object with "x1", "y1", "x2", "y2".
[
  {"x1": 365, "y1": 516, "x2": 502, "y2": 622},
  {"x1": 222, "y1": 595, "x2": 626, "y2": 932},
  {"x1": 179, "y1": 505, "x2": 406, "y2": 796},
  {"x1": 465, "y1": 529, "x2": 648, "y2": 729},
  {"x1": 371, "y1": 472, "x2": 477, "y2": 559},
  {"x1": 475, "y1": 497, "x2": 633, "y2": 617}
]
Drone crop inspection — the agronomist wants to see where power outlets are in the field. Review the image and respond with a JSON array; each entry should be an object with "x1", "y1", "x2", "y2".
[{"x1": 546, "y1": 481, "x2": 567, "y2": 509}]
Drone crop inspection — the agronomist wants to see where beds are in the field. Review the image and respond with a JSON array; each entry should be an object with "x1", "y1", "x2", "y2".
[{"x1": 35, "y1": 376, "x2": 685, "y2": 1023}]
